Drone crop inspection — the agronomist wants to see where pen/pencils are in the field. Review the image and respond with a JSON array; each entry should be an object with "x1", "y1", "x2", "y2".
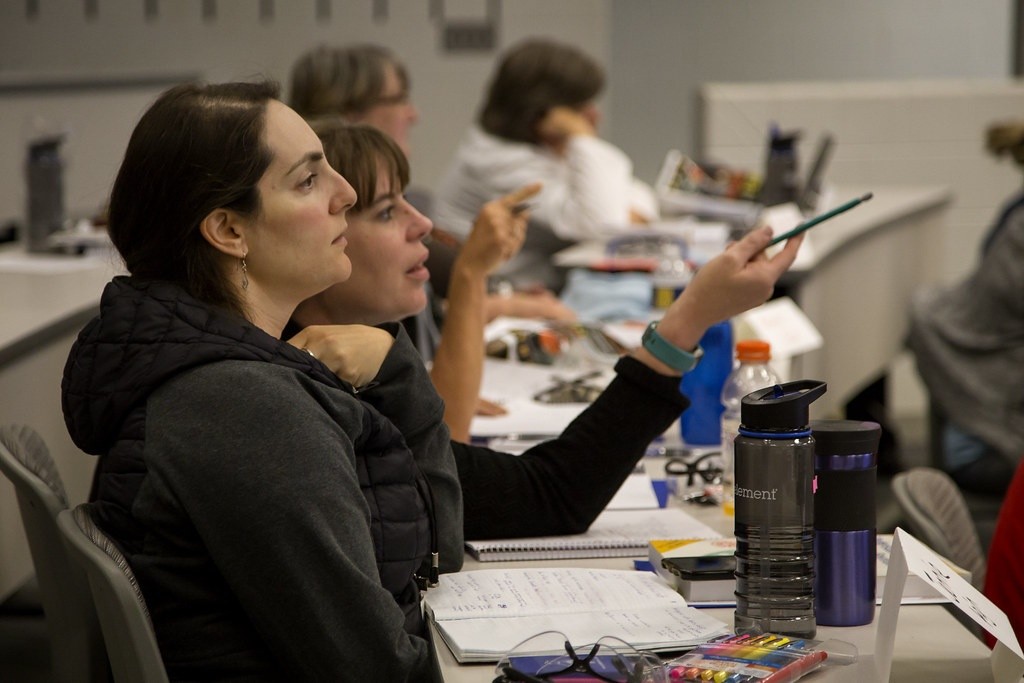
[{"x1": 755, "y1": 192, "x2": 874, "y2": 264}]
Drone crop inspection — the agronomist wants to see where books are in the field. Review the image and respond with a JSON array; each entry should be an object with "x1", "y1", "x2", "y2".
[
  {"x1": 463, "y1": 507, "x2": 725, "y2": 559},
  {"x1": 646, "y1": 537, "x2": 737, "y2": 600},
  {"x1": 420, "y1": 567, "x2": 732, "y2": 663},
  {"x1": 874, "y1": 533, "x2": 972, "y2": 600}
]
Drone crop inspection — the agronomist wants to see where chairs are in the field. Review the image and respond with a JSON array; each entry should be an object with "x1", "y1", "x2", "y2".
[{"x1": 0, "y1": 420, "x2": 168, "y2": 683}]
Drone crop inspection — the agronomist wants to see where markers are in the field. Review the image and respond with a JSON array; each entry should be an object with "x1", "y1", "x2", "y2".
[{"x1": 652, "y1": 631, "x2": 830, "y2": 683}]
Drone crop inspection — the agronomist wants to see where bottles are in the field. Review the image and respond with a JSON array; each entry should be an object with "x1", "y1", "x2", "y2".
[
  {"x1": 721, "y1": 340, "x2": 782, "y2": 538},
  {"x1": 651, "y1": 242, "x2": 694, "y2": 314}
]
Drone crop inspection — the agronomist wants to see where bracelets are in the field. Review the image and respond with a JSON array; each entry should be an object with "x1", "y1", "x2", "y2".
[{"x1": 641, "y1": 320, "x2": 704, "y2": 375}]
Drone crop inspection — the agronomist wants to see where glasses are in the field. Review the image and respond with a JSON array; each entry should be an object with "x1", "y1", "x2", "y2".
[
  {"x1": 494, "y1": 630, "x2": 666, "y2": 683},
  {"x1": 367, "y1": 92, "x2": 409, "y2": 109},
  {"x1": 535, "y1": 372, "x2": 603, "y2": 403},
  {"x1": 665, "y1": 452, "x2": 725, "y2": 486}
]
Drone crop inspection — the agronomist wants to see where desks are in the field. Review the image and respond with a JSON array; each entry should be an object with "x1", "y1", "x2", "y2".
[
  {"x1": 427, "y1": 188, "x2": 993, "y2": 683},
  {"x1": 0, "y1": 221, "x2": 130, "y2": 598}
]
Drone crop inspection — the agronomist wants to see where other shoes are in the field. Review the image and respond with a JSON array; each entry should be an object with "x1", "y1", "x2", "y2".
[{"x1": 892, "y1": 468, "x2": 986, "y2": 590}]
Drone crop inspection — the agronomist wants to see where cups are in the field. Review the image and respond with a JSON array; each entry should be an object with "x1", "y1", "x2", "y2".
[
  {"x1": 807, "y1": 419, "x2": 882, "y2": 627},
  {"x1": 732, "y1": 378, "x2": 827, "y2": 640},
  {"x1": 678, "y1": 320, "x2": 736, "y2": 444}
]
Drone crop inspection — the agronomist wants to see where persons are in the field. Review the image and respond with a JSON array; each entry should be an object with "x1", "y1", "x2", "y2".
[{"x1": 60, "y1": 36, "x2": 1023, "y2": 683}]
